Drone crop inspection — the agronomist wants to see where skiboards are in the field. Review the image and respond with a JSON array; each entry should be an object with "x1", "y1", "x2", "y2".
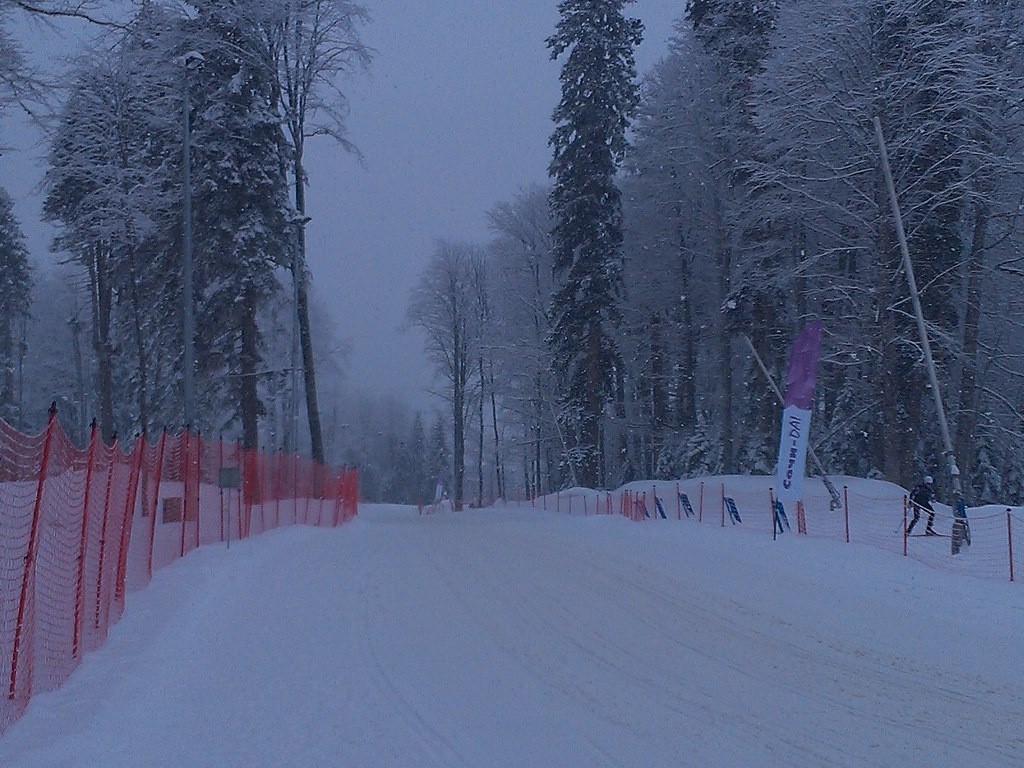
[{"x1": 908, "y1": 533, "x2": 953, "y2": 537}]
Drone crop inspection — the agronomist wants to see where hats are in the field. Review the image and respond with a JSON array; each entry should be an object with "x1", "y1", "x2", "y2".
[{"x1": 924, "y1": 476, "x2": 933, "y2": 483}]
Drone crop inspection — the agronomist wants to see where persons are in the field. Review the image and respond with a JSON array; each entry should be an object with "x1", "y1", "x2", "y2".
[{"x1": 906, "y1": 477, "x2": 937, "y2": 535}]
